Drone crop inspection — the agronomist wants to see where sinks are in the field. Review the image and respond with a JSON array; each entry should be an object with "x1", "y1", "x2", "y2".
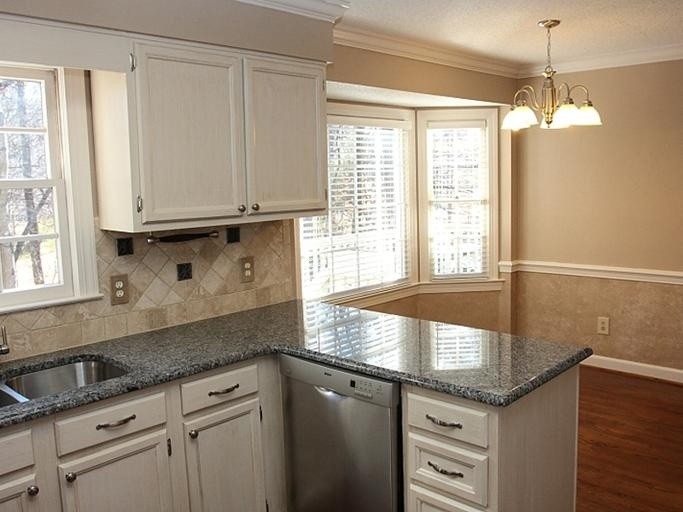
[
  {"x1": 4, "y1": 351, "x2": 132, "y2": 400},
  {"x1": 0, "y1": 388, "x2": 20, "y2": 408}
]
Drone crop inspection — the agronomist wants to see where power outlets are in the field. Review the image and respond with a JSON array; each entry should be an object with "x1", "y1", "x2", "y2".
[
  {"x1": 239, "y1": 256, "x2": 253, "y2": 282},
  {"x1": 109, "y1": 274, "x2": 129, "y2": 306}
]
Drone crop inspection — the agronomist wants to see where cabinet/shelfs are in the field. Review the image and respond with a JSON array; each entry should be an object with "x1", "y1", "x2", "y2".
[
  {"x1": 0, "y1": 351, "x2": 277, "y2": 511},
  {"x1": 89, "y1": 26, "x2": 329, "y2": 234},
  {"x1": 403, "y1": 361, "x2": 580, "y2": 511}
]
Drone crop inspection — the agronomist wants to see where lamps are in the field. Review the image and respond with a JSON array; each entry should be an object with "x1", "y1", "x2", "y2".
[{"x1": 499, "y1": 20, "x2": 602, "y2": 134}]
